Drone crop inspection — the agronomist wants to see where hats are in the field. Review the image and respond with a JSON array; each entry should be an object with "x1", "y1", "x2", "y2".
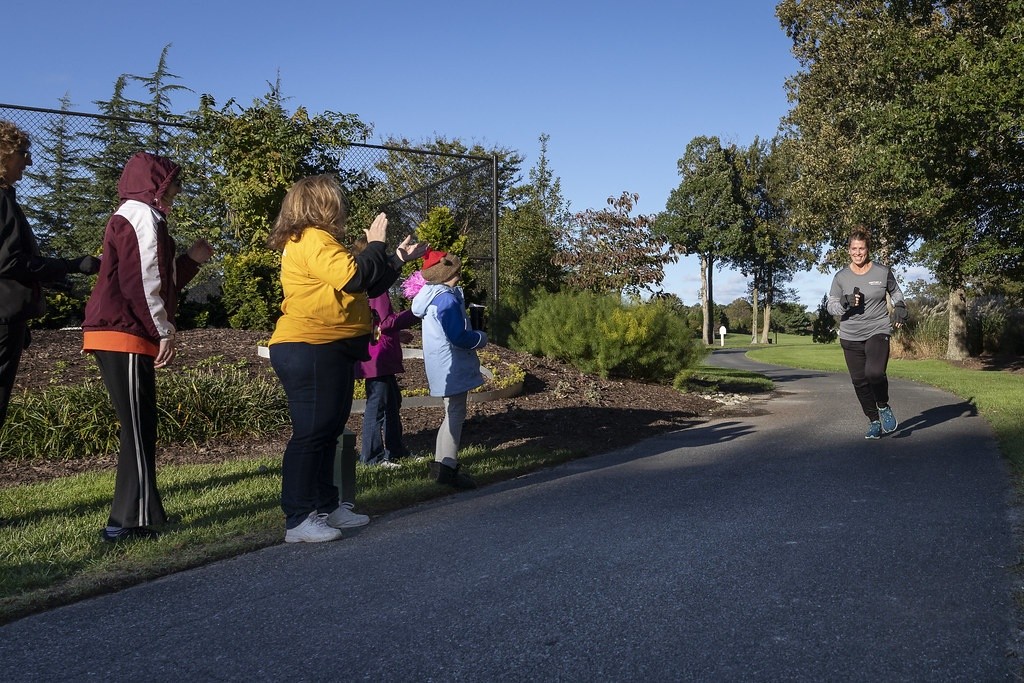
[{"x1": 422, "y1": 248, "x2": 462, "y2": 282}]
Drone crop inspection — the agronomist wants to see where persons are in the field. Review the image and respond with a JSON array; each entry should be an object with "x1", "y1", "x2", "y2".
[
  {"x1": 0, "y1": 119, "x2": 102, "y2": 437},
  {"x1": 81, "y1": 152, "x2": 211, "y2": 541},
  {"x1": 354, "y1": 236, "x2": 424, "y2": 468},
  {"x1": 827, "y1": 231, "x2": 909, "y2": 438},
  {"x1": 410, "y1": 250, "x2": 486, "y2": 482},
  {"x1": 268, "y1": 175, "x2": 418, "y2": 542}
]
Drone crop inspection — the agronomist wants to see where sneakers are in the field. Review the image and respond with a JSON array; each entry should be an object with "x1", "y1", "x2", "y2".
[
  {"x1": 877, "y1": 404, "x2": 898, "y2": 433},
  {"x1": 101, "y1": 527, "x2": 162, "y2": 544},
  {"x1": 865, "y1": 420, "x2": 882, "y2": 440},
  {"x1": 427, "y1": 460, "x2": 440, "y2": 479},
  {"x1": 436, "y1": 464, "x2": 473, "y2": 490},
  {"x1": 285, "y1": 510, "x2": 342, "y2": 544},
  {"x1": 325, "y1": 505, "x2": 370, "y2": 527}
]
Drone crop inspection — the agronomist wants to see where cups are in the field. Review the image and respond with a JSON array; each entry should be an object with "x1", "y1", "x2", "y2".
[{"x1": 469, "y1": 303, "x2": 486, "y2": 333}]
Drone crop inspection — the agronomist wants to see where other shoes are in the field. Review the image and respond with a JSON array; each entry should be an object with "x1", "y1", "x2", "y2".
[
  {"x1": 409, "y1": 454, "x2": 425, "y2": 462},
  {"x1": 380, "y1": 460, "x2": 402, "y2": 469}
]
